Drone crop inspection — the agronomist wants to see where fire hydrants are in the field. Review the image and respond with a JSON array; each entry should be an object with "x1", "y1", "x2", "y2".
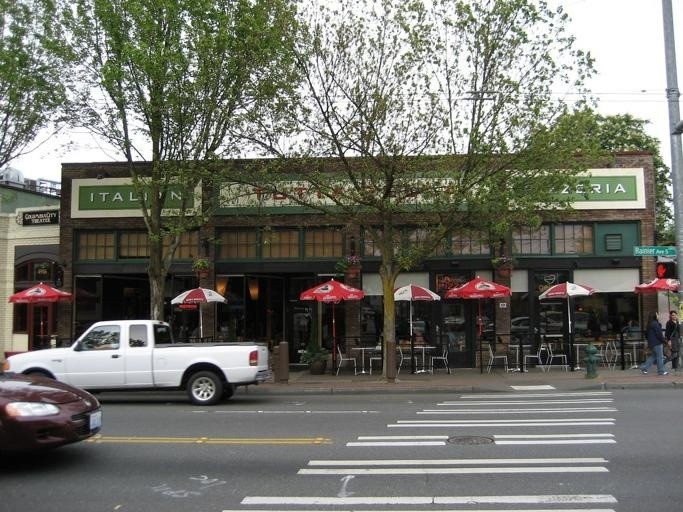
[{"x1": 580, "y1": 341, "x2": 601, "y2": 380}]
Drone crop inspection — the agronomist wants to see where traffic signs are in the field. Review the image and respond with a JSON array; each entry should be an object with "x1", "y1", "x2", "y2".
[{"x1": 631, "y1": 245, "x2": 678, "y2": 257}]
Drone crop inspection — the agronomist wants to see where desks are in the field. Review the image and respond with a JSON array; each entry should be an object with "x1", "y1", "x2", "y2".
[{"x1": 623, "y1": 341, "x2": 645, "y2": 369}]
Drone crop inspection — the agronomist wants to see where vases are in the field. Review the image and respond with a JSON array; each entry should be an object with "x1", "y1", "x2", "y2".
[
  {"x1": 497, "y1": 268, "x2": 510, "y2": 277},
  {"x1": 343, "y1": 265, "x2": 361, "y2": 279},
  {"x1": 195, "y1": 268, "x2": 207, "y2": 279}
]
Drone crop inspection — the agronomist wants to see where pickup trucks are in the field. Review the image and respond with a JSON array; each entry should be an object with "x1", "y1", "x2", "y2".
[{"x1": 3, "y1": 318, "x2": 274, "y2": 407}]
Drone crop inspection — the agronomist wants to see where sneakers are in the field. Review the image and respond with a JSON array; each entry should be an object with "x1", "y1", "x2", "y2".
[
  {"x1": 658, "y1": 371, "x2": 668, "y2": 374},
  {"x1": 640, "y1": 367, "x2": 647, "y2": 374}
]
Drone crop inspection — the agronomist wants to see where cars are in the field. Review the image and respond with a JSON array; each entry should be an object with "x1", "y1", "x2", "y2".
[
  {"x1": 0, "y1": 369, "x2": 104, "y2": 460},
  {"x1": 484, "y1": 309, "x2": 607, "y2": 338}
]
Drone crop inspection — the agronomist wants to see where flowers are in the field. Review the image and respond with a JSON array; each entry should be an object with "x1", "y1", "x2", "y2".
[
  {"x1": 190, "y1": 254, "x2": 212, "y2": 271},
  {"x1": 490, "y1": 254, "x2": 519, "y2": 270},
  {"x1": 334, "y1": 254, "x2": 362, "y2": 273}
]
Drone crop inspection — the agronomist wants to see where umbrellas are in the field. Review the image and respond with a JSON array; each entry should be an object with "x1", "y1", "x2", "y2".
[
  {"x1": 446, "y1": 274, "x2": 512, "y2": 365},
  {"x1": 300, "y1": 275, "x2": 365, "y2": 366},
  {"x1": 636, "y1": 276, "x2": 683, "y2": 312},
  {"x1": 170, "y1": 285, "x2": 227, "y2": 335},
  {"x1": 6, "y1": 280, "x2": 72, "y2": 342},
  {"x1": 392, "y1": 281, "x2": 440, "y2": 366},
  {"x1": 537, "y1": 280, "x2": 596, "y2": 361}
]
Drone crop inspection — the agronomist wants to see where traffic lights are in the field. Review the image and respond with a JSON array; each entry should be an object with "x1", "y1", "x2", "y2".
[{"x1": 654, "y1": 260, "x2": 678, "y2": 280}]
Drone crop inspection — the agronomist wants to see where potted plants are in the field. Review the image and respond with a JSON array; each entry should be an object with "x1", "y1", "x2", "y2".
[{"x1": 299, "y1": 343, "x2": 329, "y2": 375}]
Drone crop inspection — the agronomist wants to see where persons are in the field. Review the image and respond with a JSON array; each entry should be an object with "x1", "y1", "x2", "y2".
[
  {"x1": 639, "y1": 308, "x2": 667, "y2": 375},
  {"x1": 663, "y1": 309, "x2": 682, "y2": 369}
]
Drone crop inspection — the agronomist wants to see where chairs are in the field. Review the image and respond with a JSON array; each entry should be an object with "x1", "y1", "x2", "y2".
[
  {"x1": 485, "y1": 341, "x2": 631, "y2": 370},
  {"x1": 332, "y1": 343, "x2": 454, "y2": 375}
]
